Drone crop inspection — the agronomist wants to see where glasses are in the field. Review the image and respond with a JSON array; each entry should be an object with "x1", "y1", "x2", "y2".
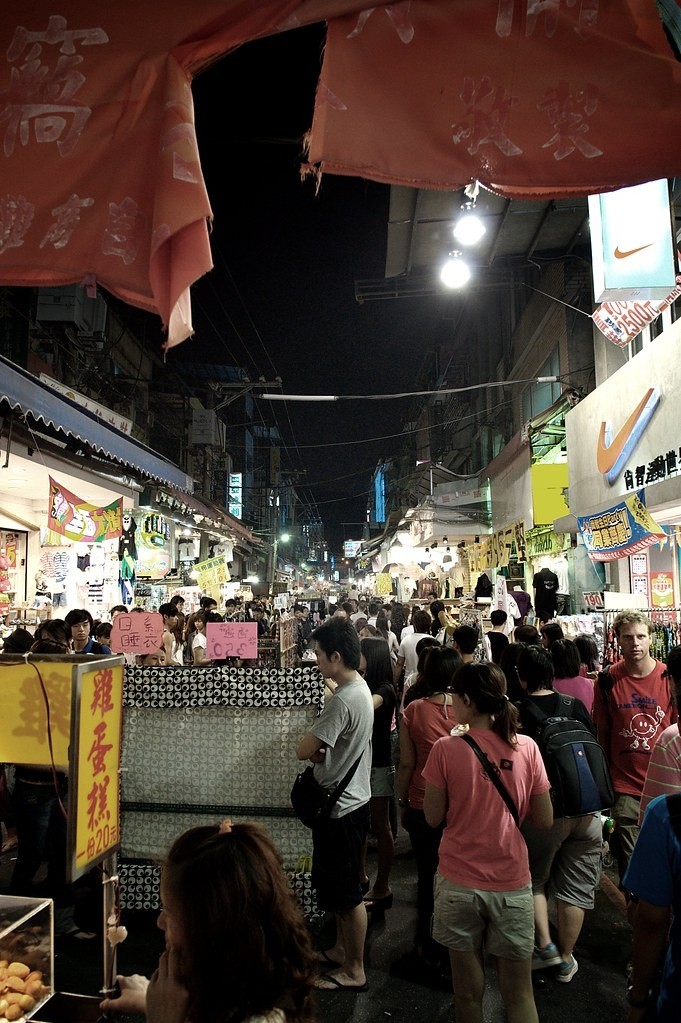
[{"x1": 447, "y1": 686, "x2": 464, "y2": 696}]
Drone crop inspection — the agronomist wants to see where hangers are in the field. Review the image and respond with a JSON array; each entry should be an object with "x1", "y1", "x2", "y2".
[{"x1": 604, "y1": 607, "x2": 681, "y2": 631}]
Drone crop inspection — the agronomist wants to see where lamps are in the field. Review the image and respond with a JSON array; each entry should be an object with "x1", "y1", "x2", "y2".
[
  {"x1": 425, "y1": 547, "x2": 430, "y2": 553},
  {"x1": 450, "y1": 192, "x2": 487, "y2": 250},
  {"x1": 438, "y1": 250, "x2": 472, "y2": 290},
  {"x1": 158, "y1": 506, "x2": 233, "y2": 540},
  {"x1": 431, "y1": 540, "x2": 438, "y2": 549},
  {"x1": 443, "y1": 535, "x2": 448, "y2": 544},
  {"x1": 458, "y1": 540, "x2": 466, "y2": 548},
  {"x1": 474, "y1": 535, "x2": 480, "y2": 543},
  {"x1": 446, "y1": 545, "x2": 451, "y2": 553}
]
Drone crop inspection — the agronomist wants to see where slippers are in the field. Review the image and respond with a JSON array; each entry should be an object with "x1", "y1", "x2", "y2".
[
  {"x1": 313, "y1": 950, "x2": 342, "y2": 966},
  {"x1": 363, "y1": 893, "x2": 394, "y2": 907},
  {"x1": 312, "y1": 973, "x2": 369, "y2": 993}
]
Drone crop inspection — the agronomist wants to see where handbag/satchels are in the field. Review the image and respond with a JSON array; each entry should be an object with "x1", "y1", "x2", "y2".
[{"x1": 291, "y1": 766, "x2": 335, "y2": 830}]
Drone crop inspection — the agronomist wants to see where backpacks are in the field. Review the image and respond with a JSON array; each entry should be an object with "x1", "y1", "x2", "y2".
[{"x1": 536, "y1": 717, "x2": 615, "y2": 817}]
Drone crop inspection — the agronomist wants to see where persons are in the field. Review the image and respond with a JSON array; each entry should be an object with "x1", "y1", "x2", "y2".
[
  {"x1": 100, "y1": 820, "x2": 316, "y2": 1023},
  {"x1": 532, "y1": 568, "x2": 559, "y2": 624},
  {"x1": 0, "y1": 585, "x2": 681, "y2": 1023},
  {"x1": 119, "y1": 515, "x2": 138, "y2": 560},
  {"x1": 510, "y1": 585, "x2": 533, "y2": 626},
  {"x1": 295, "y1": 617, "x2": 374, "y2": 993},
  {"x1": 448, "y1": 562, "x2": 467, "y2": 598},
  {"x1": 35, "y1": 565, "x2": 53, "y2": 621}
]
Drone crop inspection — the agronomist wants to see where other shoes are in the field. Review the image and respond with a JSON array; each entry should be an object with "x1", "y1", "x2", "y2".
[{"x1": 55, "y1": 925, "x2": 96, "y2": 939}]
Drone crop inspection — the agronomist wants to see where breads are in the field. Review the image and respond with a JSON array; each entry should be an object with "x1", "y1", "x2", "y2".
[{"x1": 0, "y1": 960, "x2": 44, "y2": 1020}]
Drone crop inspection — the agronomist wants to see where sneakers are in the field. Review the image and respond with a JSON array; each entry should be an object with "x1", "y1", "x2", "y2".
[
  {"x1": 557, "y1": 953, "x2": 577, "y2": 983},
  {"x1": 531, "y1": 943, "x2": 563, "y2": 970}
]
